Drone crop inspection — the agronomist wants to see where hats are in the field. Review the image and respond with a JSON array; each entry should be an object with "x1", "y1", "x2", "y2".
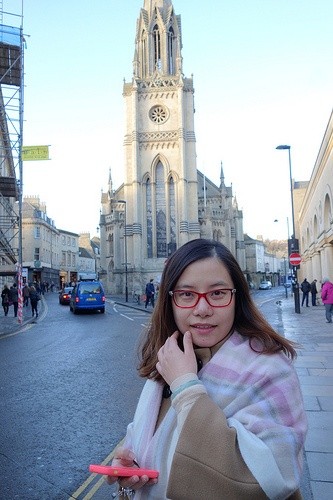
[{"x1": 323, "y1": 277, "x2": 330, "y2": 282}]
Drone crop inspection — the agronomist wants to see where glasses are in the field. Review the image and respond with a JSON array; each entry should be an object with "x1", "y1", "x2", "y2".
[{"x1": 168, "y1": 288, "x2": 236, "y2": 309}]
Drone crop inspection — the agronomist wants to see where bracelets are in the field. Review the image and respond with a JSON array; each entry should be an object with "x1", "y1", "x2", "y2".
[{"x1": 112, "y1": 459, "x2": 141, "y2": 497}]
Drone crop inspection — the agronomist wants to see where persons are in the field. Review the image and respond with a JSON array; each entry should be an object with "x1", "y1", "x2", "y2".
[
  {"x1": 300, "y1": 277, "x2": 333, "y2": 323},
  {"x1": 145, "y1": 279, "x2": 155, "y2": 311},
  {"x1": 109, "y1": 238, "x2": 307, "y2": 500},
  {"x1": 2, "y1": 279, "x2": 77, "y2": 318}
]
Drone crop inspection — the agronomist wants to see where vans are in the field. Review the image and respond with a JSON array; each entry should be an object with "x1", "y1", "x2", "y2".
[{"x1": 68, "y1": 281, "x2": 105, "y2": 314}]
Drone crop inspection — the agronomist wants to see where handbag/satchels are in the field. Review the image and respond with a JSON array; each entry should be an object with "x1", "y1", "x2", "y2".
[{"x1": 36, "y1": 294, "x2": 40, "y2": 300}]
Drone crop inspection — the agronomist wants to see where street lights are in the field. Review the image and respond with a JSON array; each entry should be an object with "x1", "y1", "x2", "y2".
[
  {"x1": 275, "y1": 145, "x2": 300, "y2": 313},
  {"x1": 118, "y1": 200, "x2": 128, "y2": 302},
  {"x1": 282, "y1": 257, "x2": 286, "y2": 282},
  {"x1": 274, "y1": 215, "x2": 290, "y2": 240}
]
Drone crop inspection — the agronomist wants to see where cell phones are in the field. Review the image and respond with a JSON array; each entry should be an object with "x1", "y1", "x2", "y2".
[{"x1": 89, "y1": 465, "x2": 158, "y2": 478}]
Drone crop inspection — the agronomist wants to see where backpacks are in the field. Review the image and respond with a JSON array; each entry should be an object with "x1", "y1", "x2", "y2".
[{"x1": 4, "y1": 294, "x2": 8, "y2": 303}]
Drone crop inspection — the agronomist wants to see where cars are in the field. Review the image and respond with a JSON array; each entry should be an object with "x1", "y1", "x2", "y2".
[
  {"x1": 58, "y1": 287, "x2": 74, "y2": 305},
  {"x1": 284, "y1": 280, "x2": 294, "y2": 288},
  {"x1": 259, "y1": 281, "x2": 272, "y2": 290}
]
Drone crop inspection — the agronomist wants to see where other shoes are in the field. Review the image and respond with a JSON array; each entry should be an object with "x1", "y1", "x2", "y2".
[{"x1": 36, "y1": 314, "x2": 38, "y2": 317}]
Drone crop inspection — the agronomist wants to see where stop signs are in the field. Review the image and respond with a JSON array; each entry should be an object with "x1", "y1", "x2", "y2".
[{"x1": 289, "y1": 254, "x2": 301, "y2": 266}]
